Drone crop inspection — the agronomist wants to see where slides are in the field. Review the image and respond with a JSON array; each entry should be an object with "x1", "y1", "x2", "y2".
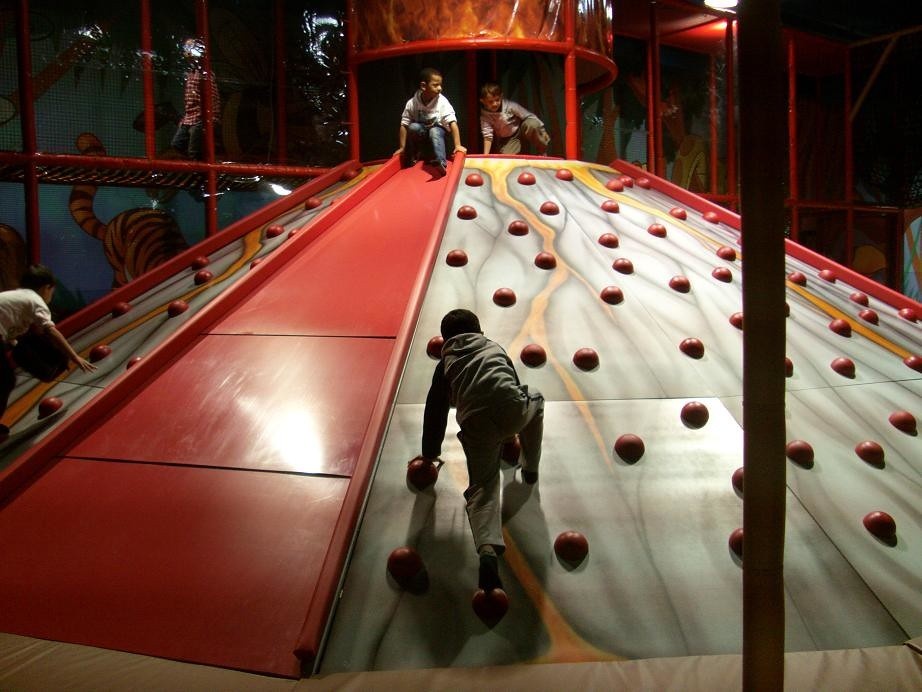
[{"x1": 0, "y1": 152, "x2": 465, "y2": 678}]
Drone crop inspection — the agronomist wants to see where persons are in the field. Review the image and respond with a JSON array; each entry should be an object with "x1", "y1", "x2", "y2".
[
  {"x1": 169, "y1": 37, "x2": 223, "y2": 187},
  {"x1": 420, "y1": 307, "x2": 544, "y2": 580},
  {"x1": 1, "y1": 266, "x2": 98, "y2": 444},
  {"x1": 480, "y1": 83, "x2": 551, "y2": 155},
  {"x1": 394, "y1": 69, "x2": 467, "y2": 176}
]
